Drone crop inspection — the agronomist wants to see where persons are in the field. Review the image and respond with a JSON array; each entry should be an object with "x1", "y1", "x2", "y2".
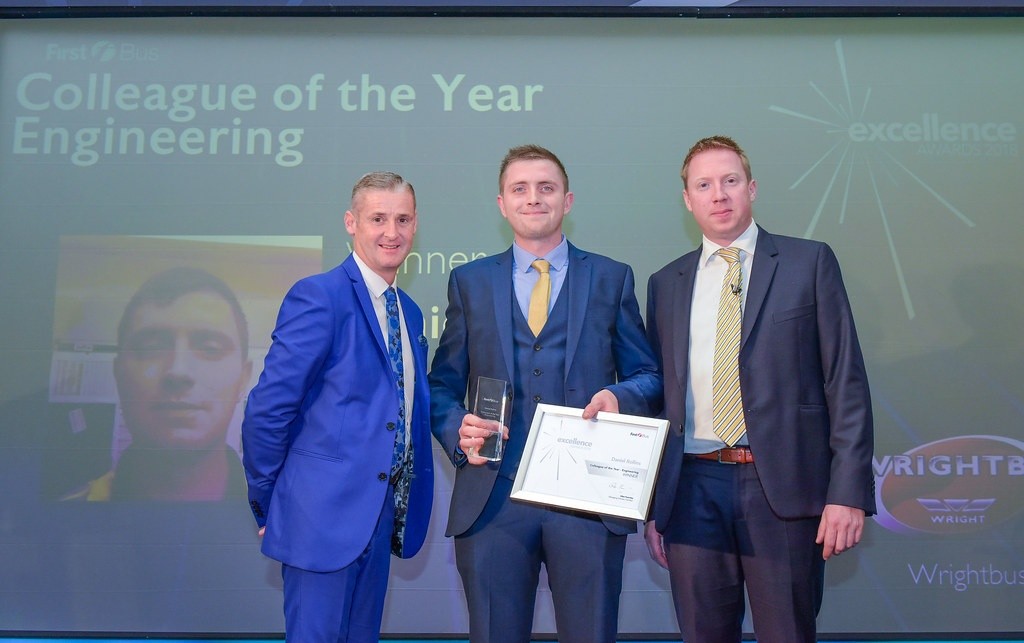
[
  {"x1": 425, "y1": 146, "x2": 663, "y2": 643},
  {"x1": 70, "y1": 264, "x2": 250, "y2": 500},
  {"x1": 241, "y1": 172, "x2": 436, "y2": 643},
  {"x1": 644, "y1": 137, "x2": 878, "y2": 643}
]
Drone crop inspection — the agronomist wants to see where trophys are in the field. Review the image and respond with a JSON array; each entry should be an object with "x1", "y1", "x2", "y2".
[{"x1": 469, "y1": 376, "x2": 510, "y2": 463}]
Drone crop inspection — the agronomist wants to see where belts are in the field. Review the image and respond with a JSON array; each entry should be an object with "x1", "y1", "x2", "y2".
[{"x1": 684, "y1": 448, "x2": 753, "y2": 464}]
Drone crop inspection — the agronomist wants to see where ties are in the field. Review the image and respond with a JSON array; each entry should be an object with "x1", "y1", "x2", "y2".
[
  {"x1": 383, "y1": 286, "x2": 405, "y2": 478},
  {"x1": 714, "y1": 246, "x2": 747, "y2": 449},
  {"x1": 527, "y1": 258, "x2": 551, "y2": 339}
]
